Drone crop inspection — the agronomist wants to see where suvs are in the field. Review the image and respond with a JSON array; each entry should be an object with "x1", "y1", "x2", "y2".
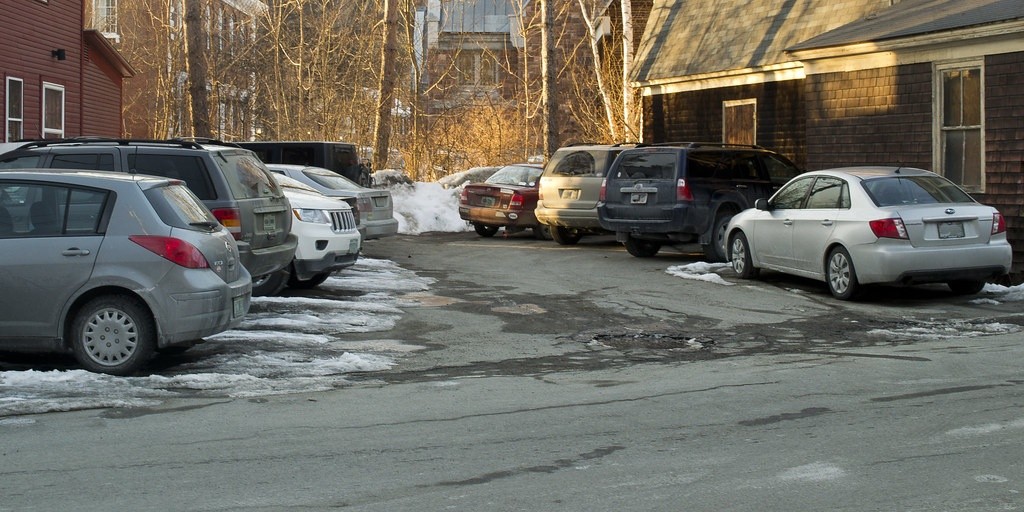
[
  {"x1": 598, "y1": 141, "x2": 805, "y2": 263},
  {"x1": 535, "y1": 141, "x2": 642, "y2": 246},
  {"x1": 1, "y1": 136, "x2": 299, "y2": 288}
]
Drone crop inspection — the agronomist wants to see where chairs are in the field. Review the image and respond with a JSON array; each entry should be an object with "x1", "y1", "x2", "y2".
[
  {"x1": 0, "y1": 205, "x2": 20, "y2": 238},
  {"x1": 26, "y1": 201, "x2": 58, "y2": 235}
]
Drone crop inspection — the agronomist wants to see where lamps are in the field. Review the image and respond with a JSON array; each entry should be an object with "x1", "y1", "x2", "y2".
[{"x1": 51, "y1": 48, "x2": 66, "y2": 61}]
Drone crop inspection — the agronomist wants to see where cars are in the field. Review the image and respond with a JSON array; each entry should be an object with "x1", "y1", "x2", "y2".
[
  {"x1": 459, "y1": 163, "x2": 554, "y2": 240},
  {"x1": 724, "y1": 166, "x2": 1012, "y2": 300},
  {"x1": 197, "y1": 142, "x2": 399, "y2": 297},
  {"x1": 0, "y1": 168, "x2": 252, "y2": 374}
]
